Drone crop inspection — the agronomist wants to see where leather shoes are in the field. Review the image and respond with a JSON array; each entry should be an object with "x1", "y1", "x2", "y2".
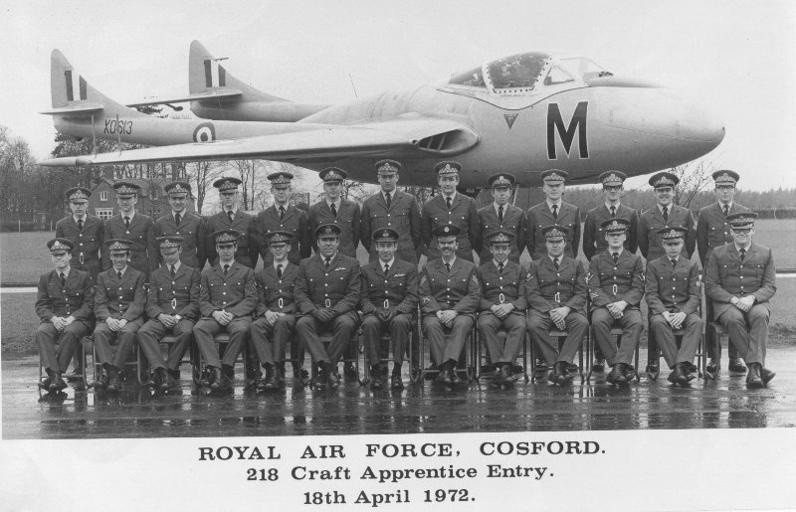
[
  {"x1": 39, "y1": 364, "x2": 285, "y2": 395},
  {"x1": 535, "y1": 358, "x2": 775, "y2": 390},
  {"x1": 290, "y1": 363, "x2": 527, "y2": 392}
]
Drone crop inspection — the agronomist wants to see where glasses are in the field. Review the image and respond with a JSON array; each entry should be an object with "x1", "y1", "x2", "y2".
[
  {"x1": 716, "y1": 184, "x2": 736, "y2": 191},
  {"x1": 601, "y1": 185, "x2": 622, "y2": 191},
  {"x1": 732, "y1": 229, "x2": 752, "y2": 236}
]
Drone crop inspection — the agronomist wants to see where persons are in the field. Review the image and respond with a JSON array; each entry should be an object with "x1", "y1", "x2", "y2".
[{"x1": 34, "y1": 159, "x2": 777, "y2": 390}]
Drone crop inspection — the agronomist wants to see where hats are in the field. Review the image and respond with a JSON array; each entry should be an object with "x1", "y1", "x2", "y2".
[
  {"x1": 66, "y1": 187, "x2": 92, "y2": 204},
  {"x1": 47, "y1": 237, "x2": 73, "y2": 256},
  {"x1": 155, "y1": 235, "x2": 182, "y2": 254},
  {"x1": 315, "y1": 210, "x2": 758, "y2": 247},
  {"x1": 212, "y1": 229, "x2": 240, "y2": 246},
  {"x1": 112, "y1": 181, "x2": 140, "y2": 198},
  {"x1": 320, "y1": 158, "x2": 740, "y2": 192},
  {"x1": 264, "y1": 230, "x2": 294, "y2": 247},
  {"x1": 266, "y1": 172, "x2": 293, "y2": 189},
  {"x1": 165, "y1": 181, "x2": 191, "y2": 199},
  {"x1": 105, "y1": 238, "x2": 134, "y2": 254},
  {"x1": 213, "y1": 177, "x2": 243, "y2": 193}
]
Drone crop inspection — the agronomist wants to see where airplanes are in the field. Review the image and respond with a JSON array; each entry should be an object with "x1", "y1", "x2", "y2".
[{"x1": 36, "y1": 38, "x2": 730, "y2": 200}]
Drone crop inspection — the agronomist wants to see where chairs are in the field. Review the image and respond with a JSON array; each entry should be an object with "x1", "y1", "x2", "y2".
[{"x1": 36, "y1": 279, "x2": 763, "y2": 393}]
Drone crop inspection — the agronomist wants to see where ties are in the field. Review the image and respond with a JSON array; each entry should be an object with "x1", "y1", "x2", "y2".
[
  {"x1": 78, "y1": 218, "x2": 83, "y2": 231},
  {"x1": 227, "y1": 211, "x2": 234, "y2": 224},
  {"x1": 331, "y1": 192, "x2": 735, "y2": 222},
  {"x1": 223, "y1": 264, "x2": 230, "y2": 276},
  {"x1": 118, "y1": 271, "x2": 122, "y2": 280},
  {"x1": 275, "y1": 263, "x2": 283, "y2": 281},
  {"x1": 125, "y1": 216, "x2": 130, "y2": 229},
  {"x1": 170, "y1": 265, "x2": 177, "y2": 280},
  {"x1": 60, "y1": 273, "x2": 65, "y2": 287},
  {"x1": 322, "y1": 245, "x2": 749, "y2": 272},
  {"x1": 175, "y1": 213, "x2": 181, "y2": 227},
  {"x1": 279, "y1": 205, "x2": 286, "y2": 221}
]
